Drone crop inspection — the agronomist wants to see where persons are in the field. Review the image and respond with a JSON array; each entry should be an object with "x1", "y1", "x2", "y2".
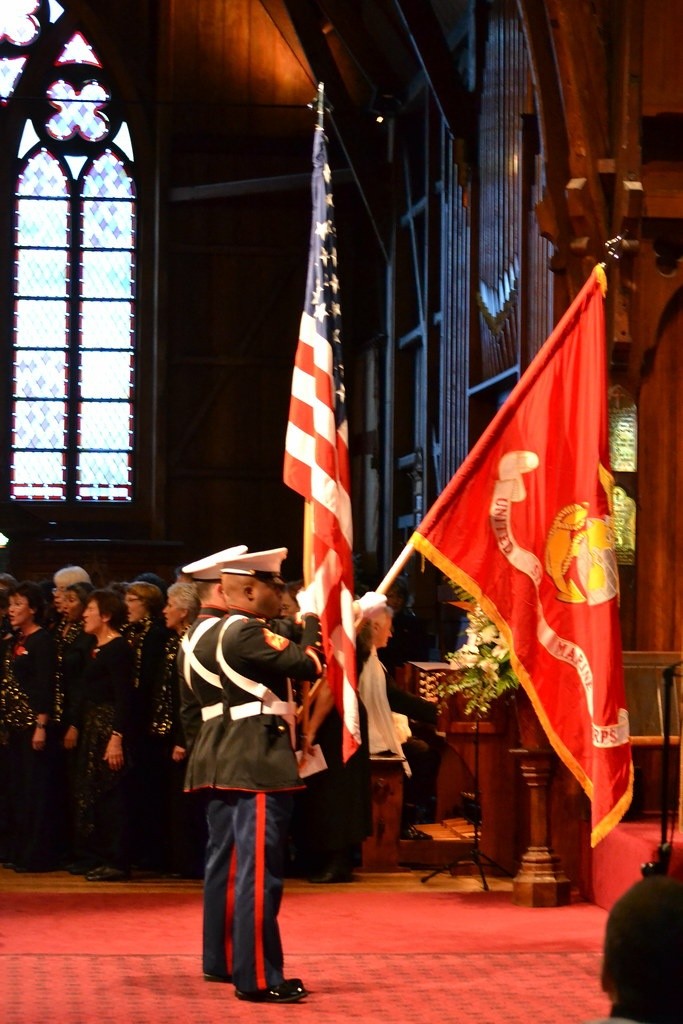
[
  {"x1": 359, "y1": 607, "x2": 436, "y2": 840},
  {"x1": 214, "y1": 548, "x2": 325, "y2": 1002},
  {"x1": 0, "y1": 566, "x2": 309, "y2": 881},
  {"x1": 179, "y1": 545, "x2": 251, "y2": 980}
]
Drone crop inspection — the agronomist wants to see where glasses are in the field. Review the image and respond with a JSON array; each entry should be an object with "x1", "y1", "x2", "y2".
[
  {"x1": 51, "y1": 588, "x2": 68, "y2": 594},
  {"x1": 124, "y1": 598, "x2": 141, "y2": 605}
]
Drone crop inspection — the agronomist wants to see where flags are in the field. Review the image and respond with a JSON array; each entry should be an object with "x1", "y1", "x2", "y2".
[
  {"x1": 281, "y1": 129, "x2": 351, "y2": 762},
  {"x1": 408, "y1": 265, "x2": 631, "y2": 848}
]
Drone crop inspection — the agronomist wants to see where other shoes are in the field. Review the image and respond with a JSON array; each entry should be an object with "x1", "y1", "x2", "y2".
[
  {"x1": 85, "y1": 864, "x2": 131, "y2": 881},
  {"x1": 309, "y1": 855, "x2": 352, "y2": 882},
  {"x1": 399, "y1": 824, "x2": 434, "y2": 839},
  {"x1": 203, "y1": 972, "x2": 236, "y2": 982},
  {"x1": 2, "y1": 862, "x2": 16, "y2": 869},
  {"x1": 15, "y1": 861, "x2": 55, "y2": 872},
  {"x1": 234, "y1": 979, "x2": 308, "y2": 1002}
]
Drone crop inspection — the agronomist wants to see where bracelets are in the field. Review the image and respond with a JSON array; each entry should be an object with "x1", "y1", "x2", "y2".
[{"x1": 36, "y1": 723, "x2": 47, "y2": 729}]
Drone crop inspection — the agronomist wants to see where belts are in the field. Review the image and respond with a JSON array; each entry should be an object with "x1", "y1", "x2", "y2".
[
  {"x1": 201, "y1": 701, "x2": 225, "y2": 722},
  {"x1": 229, "y1": 701, "x2": 297, "y2": 721}
]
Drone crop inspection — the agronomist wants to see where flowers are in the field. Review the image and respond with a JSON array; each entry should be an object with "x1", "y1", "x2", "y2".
[{"x1": 437, "y1": 601, "x2": 519, "y2": 716}]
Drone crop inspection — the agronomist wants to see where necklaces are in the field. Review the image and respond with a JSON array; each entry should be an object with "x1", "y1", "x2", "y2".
[{"x1": 107, "y1": 633, "x2": 114, "y2": 639}]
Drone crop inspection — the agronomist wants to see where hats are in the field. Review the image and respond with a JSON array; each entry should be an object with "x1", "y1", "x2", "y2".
[
  {"x1": 216, "y1": 547, "x2": 289, "y2": 585},
  {"x1": 182, "y1": 543, "x2": 248, "y2": 583}
]
